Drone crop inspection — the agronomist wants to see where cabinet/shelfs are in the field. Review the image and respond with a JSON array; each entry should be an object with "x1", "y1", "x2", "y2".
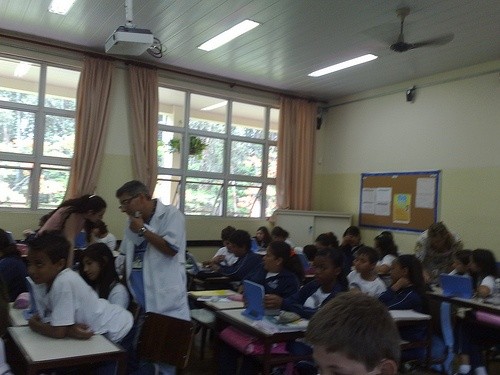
[{"x1": 274, "y1": 209, "x2": 352, "y2": 248}]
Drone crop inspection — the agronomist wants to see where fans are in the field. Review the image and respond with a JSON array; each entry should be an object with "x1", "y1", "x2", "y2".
[{"x1": 361, "y1": 0, "x2": 455, "y2": 54}]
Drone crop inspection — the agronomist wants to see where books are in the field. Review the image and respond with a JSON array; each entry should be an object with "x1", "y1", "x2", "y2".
[
  {"x1": 190, "y1": 290, "x2": 233, "y2": 298},
  {"x1": 389, "y1": 310, "x2": 430, "y2": 320},
  {"x1": 480, "y1": 296, "x2": 500, "y2": 305},
  {"x1": 263, "y1": 315, "x2": 310, "y2": 333}
]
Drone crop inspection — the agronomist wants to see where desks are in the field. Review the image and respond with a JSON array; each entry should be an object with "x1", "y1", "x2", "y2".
[{"x1": 7, "y1": 249, "x2": 500, "y2": 375}]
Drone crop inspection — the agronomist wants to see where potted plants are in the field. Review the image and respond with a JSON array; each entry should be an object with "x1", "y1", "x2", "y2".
[{"x1": 167, "y1": 135, "x2": 209, "y2": 161}]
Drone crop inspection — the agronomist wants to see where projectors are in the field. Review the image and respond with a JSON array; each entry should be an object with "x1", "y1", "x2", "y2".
[{"x1": 104, "y1": 26, "x2": 154, "y2": 55}]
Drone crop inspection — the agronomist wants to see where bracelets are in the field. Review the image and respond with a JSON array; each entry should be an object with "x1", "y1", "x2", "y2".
[{"x1": 137, "y1": 226, "x2": 147, "y2": 233}]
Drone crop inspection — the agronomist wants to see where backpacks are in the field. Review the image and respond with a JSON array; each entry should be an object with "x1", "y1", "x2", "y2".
[
  {"x1": 423, "y1": 300, "x2": 456, "y2": 375},
  {"x1": 220, "y1": 325, "x2": 294, "y2": 375}
]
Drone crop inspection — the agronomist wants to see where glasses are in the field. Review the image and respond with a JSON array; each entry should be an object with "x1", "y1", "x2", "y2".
[{"x1": 121, "y1": 193, "x2": 140, "y2": 206}]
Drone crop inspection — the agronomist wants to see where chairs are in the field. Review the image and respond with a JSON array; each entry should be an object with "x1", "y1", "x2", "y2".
[{"x1": 132, "y1": 312, "x2": 192, "y2": 375}]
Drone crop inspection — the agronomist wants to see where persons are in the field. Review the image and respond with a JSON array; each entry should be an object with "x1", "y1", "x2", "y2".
[
  {"x1": 185, "y1": 221, "x2": 500, "y2": 375},
  {"x1": 115, "y1": 181, "x2": 191, "y2": 375},
  {"x1": 0, "y1": 194, "x2": 159, "y2": 375}
]
